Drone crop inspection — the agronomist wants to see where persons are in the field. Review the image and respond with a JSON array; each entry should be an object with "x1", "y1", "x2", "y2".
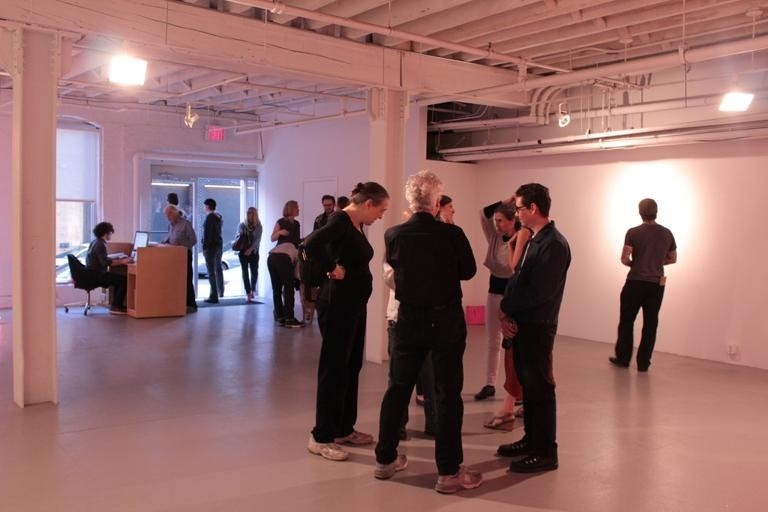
[
  {"x1": 166, "y1": 192, "x2": 192, "y2": 222},
  {"x1": 475, "y1": 182, "x2": 571, "y2": 474},
  {"x1": 266, "y1": 195, "x2": 350, "y2": 328},
  {"x1": 608, "y1": 198, "x2": 677, "y2": 372},
  {"x1": 232, "y1": 207, "x2": 262, "y2": 304},
  {"x1": 299, "y1": 180, "x2": 389, "y2": 462},
  {"x1": 87, "y1": 222, "x2": 133, "y2": 314},
  {"x1": 373, "y1": 169, "x2": 483, "y2": 492},
  {"x1": 160, "y1": 205, "x2": 198, "y2": 312},
  {"x1": 201, "y1": 198, "x2": 223, "y2": 303}
]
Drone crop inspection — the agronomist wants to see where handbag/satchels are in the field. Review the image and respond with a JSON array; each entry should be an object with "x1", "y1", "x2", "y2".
[
  {"x1": 296, "y1": 238, "x2": 333, "y2": 303},
  {"x1": 232, "y1": 236, "x2": 243, "y2": 251}
]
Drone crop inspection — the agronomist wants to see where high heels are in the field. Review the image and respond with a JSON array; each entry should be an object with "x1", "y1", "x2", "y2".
[
  {"x1": 515, "y1": 404, "x2": 524, "y2": 417},
  {"x1": 484, "y1": 411, "x2": 516, "y2": 433}
]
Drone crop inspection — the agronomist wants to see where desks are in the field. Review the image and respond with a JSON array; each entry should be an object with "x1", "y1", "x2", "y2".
[{"x1": 105, "y1": 242, "x2": 188, "y2": 319}]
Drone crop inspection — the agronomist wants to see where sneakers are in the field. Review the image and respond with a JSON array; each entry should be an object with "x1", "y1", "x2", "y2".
[
  {"x1": 474, "y1": 386, "x2": 497, "y2": 401},
  {"x1": 285, "y1": 318, "x2": 305, "y2": 329},
  {"x1": 109, "y1": 305, "x2": 127, "y2": 315},
  {"x1": 373, "y1": 452, "x2": 408, "y2": 480},
  {"x1": 435, "y1": 465, "x2": 486, "y2": 495},
  {"x1": 334, "y1": 429, "x2": 374, "y2": 446},
  {"x1": 276, "y1": 317, "x2": 284, "y2": 326},
  {"x1": 307, "y1": 431, "x2": 348, "y2": 462}
]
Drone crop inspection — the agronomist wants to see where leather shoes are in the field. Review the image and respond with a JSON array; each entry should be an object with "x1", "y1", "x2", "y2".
[
  {"x1": 187, "y1": 305, "x2": 198, "y2": 313},
  {"x1": 609, "y1": 356, "x2": 629, "y2": 367},
  {"x1": 416, "y1": 395, "x2": 424, "y2": 405},
  {"x1": 509, "y1": 445, "x2": 559, "y2": 473},
  {"x1": 638, "y1": 365, "x2": 649, "y2": 372},
  {"x1": 204, "y1": 298, "x2": 220, "y2": 303},
  {"x1": 497, "y1": 437, "x2": 530, "y2": 456}
]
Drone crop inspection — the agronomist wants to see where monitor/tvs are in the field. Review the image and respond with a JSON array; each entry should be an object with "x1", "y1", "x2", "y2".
[{"x1": 130, "y1": 231, "x2": 149, "y2": 263}]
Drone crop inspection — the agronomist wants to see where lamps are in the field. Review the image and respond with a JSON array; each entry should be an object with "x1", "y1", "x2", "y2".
[
  {"x1": 718, "y1": 86, "x2": 755, "y2": 112},
  {"x1": 556, "y1": 103, "x2": 570, "y2": 127}
]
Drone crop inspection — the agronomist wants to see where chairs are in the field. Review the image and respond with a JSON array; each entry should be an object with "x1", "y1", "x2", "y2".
[{"x1": 64, "y1": 254, "x2": 107, "y2": 315}]
